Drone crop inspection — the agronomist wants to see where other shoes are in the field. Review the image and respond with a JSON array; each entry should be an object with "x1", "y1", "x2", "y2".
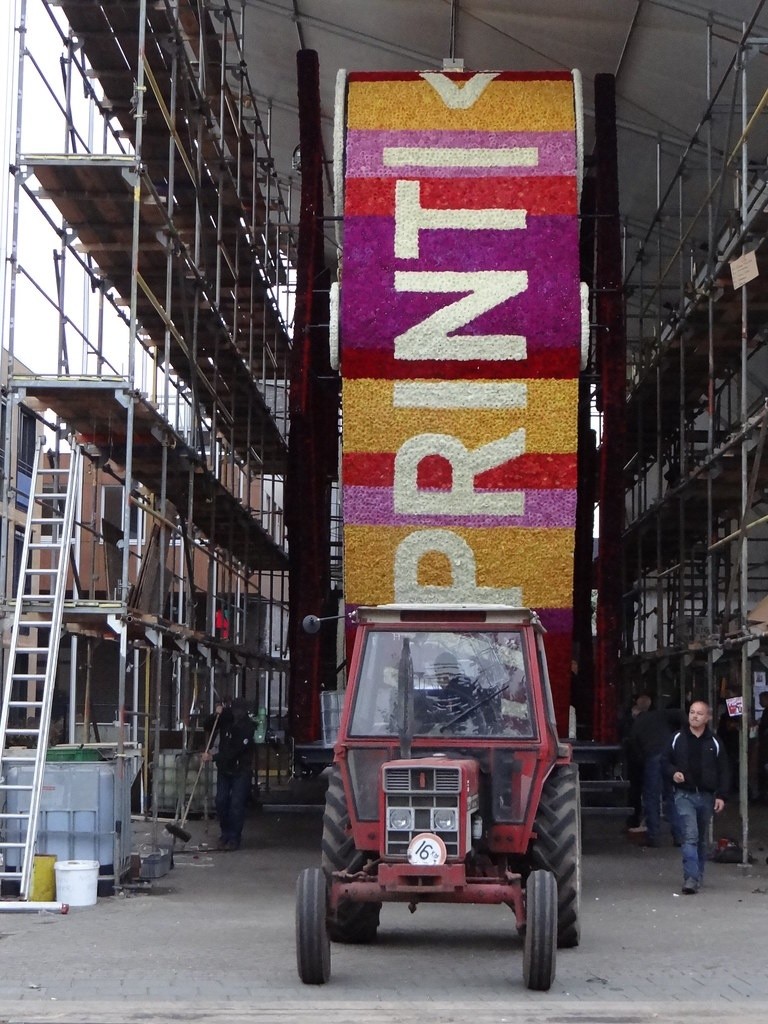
[
  {"x1": 698, "y1": 874, "x2": 703, "y2": 887},
  {"x1": 682, "y1": 877, "x2": 698, "y2": 893},
  {"x1": 215, "y1": 835, "x2": 229, "y2": 846},
  {"x1": 224, "y1": 839, "x2": 241, "y2": 850},
  {"x1": 672, "y1": 842, "x2": 681, "y2": 847},
  {"x1": 639, "y1": 841, "x2": 660, "y2": 848}
]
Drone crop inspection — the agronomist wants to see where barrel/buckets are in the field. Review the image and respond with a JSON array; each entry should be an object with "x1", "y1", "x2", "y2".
[
  {"x1": 54, "y1": 859, "x2": 100, "y2": 906},
  {"x1": 30, "y1": 855, "x2": 58, "y2": 902}
]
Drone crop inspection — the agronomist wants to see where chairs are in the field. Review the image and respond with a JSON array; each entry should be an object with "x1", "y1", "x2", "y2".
[{"x1": 415, "y1": 687, "x2": 501, "y2": 731}]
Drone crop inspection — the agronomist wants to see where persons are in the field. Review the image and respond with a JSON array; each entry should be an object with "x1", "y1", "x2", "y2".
[
  {"x1": 619, "y1": 696, "x2": 670, "y2": 832},
  {"x1": 203, "y1": 697, "x2": 252, "y2": 852},
  {"x1": 717, "y1": 711, "x2": 740, "y2": 806},
  {"x1": 568, "y1": 657, "x2": 592, "y2": 740},
  {"x1": 660, "y1": 701, "x2": 729, "y2": 894},
  {"x1": 752, "y1": 691, "x2": 768, "y2": 807},
  {"x1": 419, "y1": 652, "x2": 498, "y2": 737}
]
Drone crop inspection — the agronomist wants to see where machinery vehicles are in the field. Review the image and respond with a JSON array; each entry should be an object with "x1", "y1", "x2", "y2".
[{"x1": 264, "y1": 602, "x2": 634, "y2": 990}]
